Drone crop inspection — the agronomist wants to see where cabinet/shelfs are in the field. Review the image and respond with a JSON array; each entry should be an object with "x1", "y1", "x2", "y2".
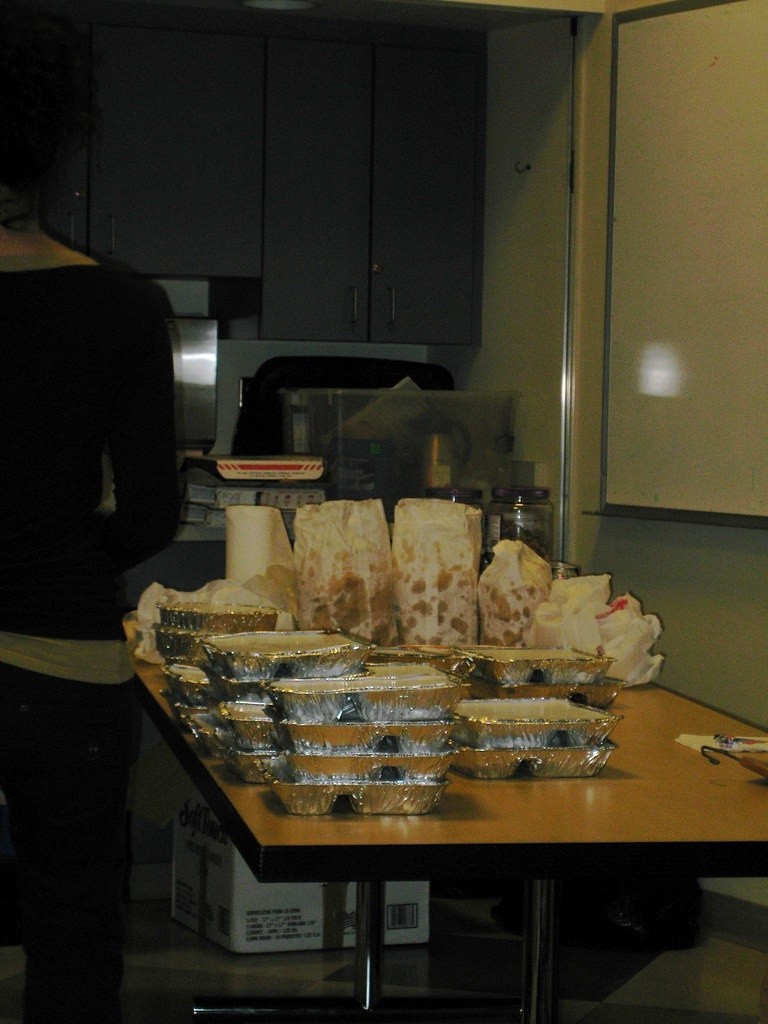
[{"x1": 38, "y1": 6, "x2": 487, "y2": 345}]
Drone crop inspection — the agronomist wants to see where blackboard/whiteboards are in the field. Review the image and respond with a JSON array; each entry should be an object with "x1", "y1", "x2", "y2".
[{"x1": 599, "y1": 0, "x2": 768, "y2": 530}]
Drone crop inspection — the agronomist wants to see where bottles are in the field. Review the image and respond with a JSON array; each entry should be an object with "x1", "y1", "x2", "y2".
[
  {"x1": 428, "y1": 488, "x2": 487, "y2": 560},
  {"x1": 490, "y1": 487, "x2": 554, "y2": 562}
]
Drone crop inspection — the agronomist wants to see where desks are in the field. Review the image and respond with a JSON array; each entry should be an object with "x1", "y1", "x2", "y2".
[{"x1": 125, "y1": 640, "x2": 768, "y2": 1024}]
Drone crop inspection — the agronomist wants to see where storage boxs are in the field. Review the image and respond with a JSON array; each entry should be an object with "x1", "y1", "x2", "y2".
[
  {"x1": 178, "y1": 456, "x2": 328, "y2": 527},
  {"x1": 283, "y1": 388, "x2": 519, "y2": 505},
  {"x1": 168, "y1": 766, "x2": 431, "y2": 954}
]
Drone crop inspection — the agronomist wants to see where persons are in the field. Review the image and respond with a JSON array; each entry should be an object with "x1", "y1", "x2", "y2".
[{"x1": 0, "y1": 0, "x2": 183, "y2": 1024}]
